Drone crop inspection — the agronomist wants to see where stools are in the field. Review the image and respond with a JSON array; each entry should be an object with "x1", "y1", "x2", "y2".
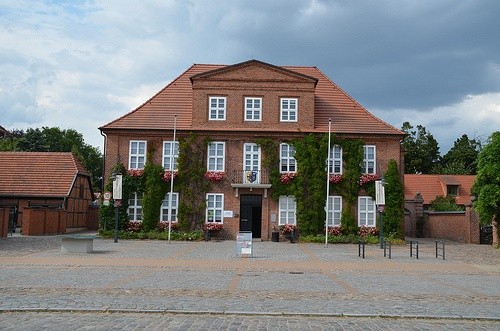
[{"x1": 56, "y1": 235, "x2": 94, "y2": 253}]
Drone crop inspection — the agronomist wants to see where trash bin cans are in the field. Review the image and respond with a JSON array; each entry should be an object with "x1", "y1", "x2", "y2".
[{"x1": 272, "y1": 231, "x2": 280, "y2": 242}]
